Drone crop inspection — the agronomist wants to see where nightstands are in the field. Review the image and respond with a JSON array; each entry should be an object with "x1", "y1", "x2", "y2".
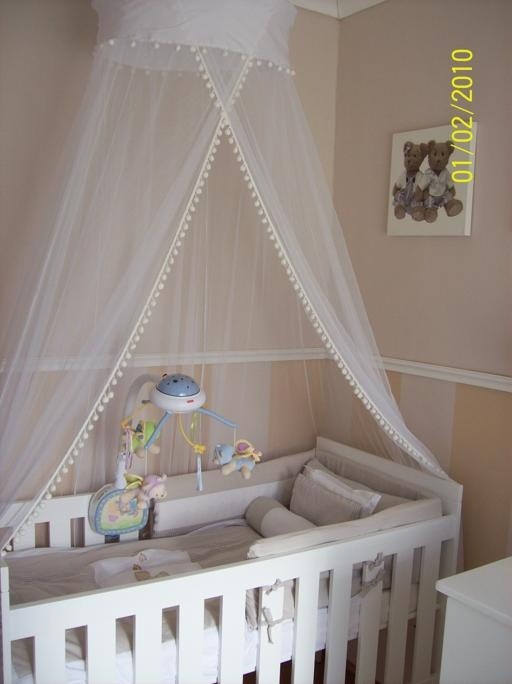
[{"x1": 434, "y1": 550, "x2": 512, "y2": 684}]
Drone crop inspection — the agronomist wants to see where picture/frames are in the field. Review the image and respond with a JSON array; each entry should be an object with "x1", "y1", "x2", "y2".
[{"x1": 383, "y1": 118, "x2": 480, "y2": 239}]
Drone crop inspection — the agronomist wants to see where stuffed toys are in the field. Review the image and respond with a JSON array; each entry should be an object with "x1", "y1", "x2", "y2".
[{"x1": 87, "y1": 371, "x2": 263, "y2": 535}]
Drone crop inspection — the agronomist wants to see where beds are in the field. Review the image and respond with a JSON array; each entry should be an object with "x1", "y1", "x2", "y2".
[{"x1": 0, "y1": 436, "x2": 464, "y2": 684}]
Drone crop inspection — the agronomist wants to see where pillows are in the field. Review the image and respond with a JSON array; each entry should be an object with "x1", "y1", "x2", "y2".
[{"x1": 244, "y1": 457, "x2": 415, "y2": 537}]
